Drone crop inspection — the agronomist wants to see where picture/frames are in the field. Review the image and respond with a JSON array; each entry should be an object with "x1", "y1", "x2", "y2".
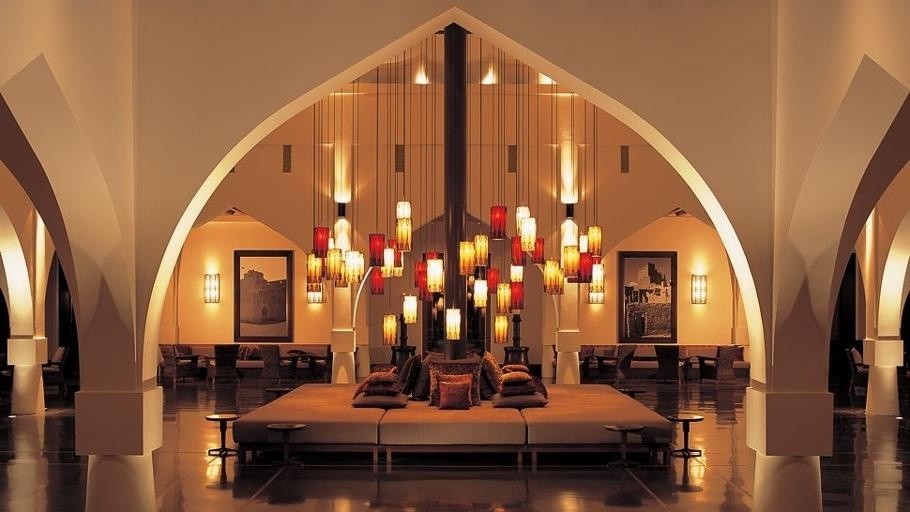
[
  {"x1": 234, "y1": 250, "x2": 295, "y2": 342},
  {"x1": 617, "y1": 251, "x2": 677, "y2": 343}
]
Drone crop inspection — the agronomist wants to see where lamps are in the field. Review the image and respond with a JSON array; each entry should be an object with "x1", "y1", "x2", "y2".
[
  {"x1": 691, "y1": 275, "x2": 707, "y2": 304},
  {"x1": 204, "y1": 274, "x2": 220, "y2": 303}
]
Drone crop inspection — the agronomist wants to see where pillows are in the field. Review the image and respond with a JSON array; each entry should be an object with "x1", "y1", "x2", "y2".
[{"x1": 352, "y1": 351, "x2": 551, "y2": 408}]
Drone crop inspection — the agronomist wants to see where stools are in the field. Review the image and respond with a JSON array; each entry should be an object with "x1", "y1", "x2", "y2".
[
  {"x1": 205, "y1": 457, "x2": 233, "y2": 489},
  {"x1": 668, "y1": 414, "x2": 704, "y2": 457},
  {"x1": 676, "y1": 458, "x2": 702, "y2": 492},
  {"x1": 205, "y1": 414, "x2": 238, "y2": 457}
]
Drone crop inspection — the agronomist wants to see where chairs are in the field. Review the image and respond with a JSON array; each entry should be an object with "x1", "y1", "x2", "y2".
[
  {"x1": 158, "y1": 344, "x2": 331, "y2": 390},
  {"x1": 580, "y1": 345, "x2": 744, "y2": 385},
  {"x1": 43, "y1": 346, "x2": 69, "y2": 393},
  {"x1": 847, "y1": 346, "x2": 868, "y2": 405}
]
